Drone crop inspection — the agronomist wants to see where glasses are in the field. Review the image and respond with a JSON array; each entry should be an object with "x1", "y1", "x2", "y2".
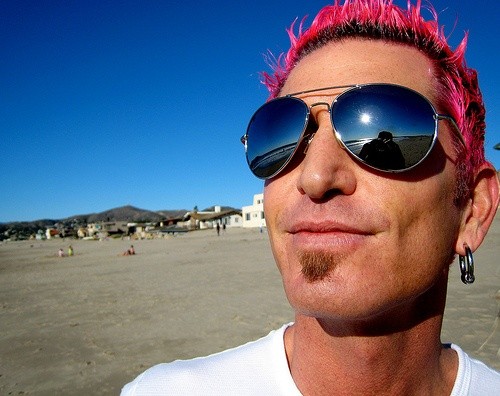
[{"x1": 240, "y1": 82, "x2": 464, "y2": 179}]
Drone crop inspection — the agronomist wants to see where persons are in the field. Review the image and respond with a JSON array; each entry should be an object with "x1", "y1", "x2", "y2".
[
  {"x1": 128, "y1": 0, "x2": 499, "y2": 396},
  {"x1": 3, "y1": 216, "x2": 237, "y2": 257},
  {"x1": 358, "y1": 131, "x2": 405, "y2": 170}
]
